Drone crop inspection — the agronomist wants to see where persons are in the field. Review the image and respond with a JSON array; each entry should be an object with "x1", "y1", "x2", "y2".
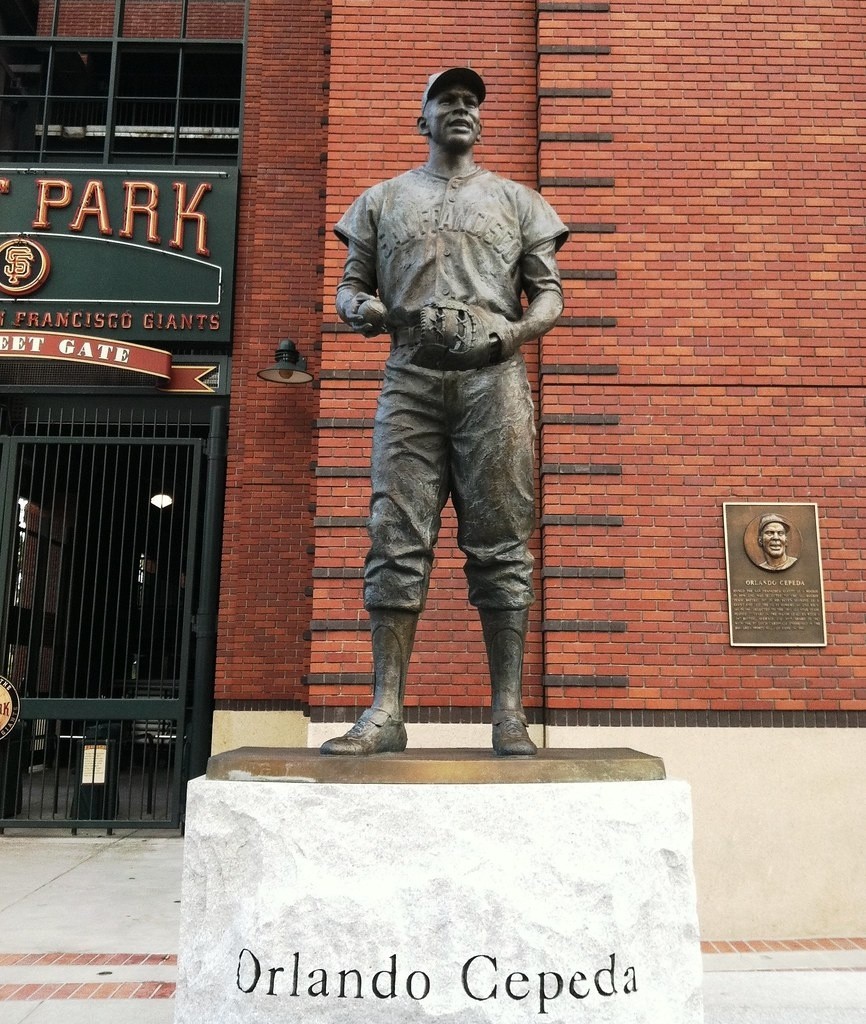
[
  {"x1": 757, "y1": 515, "x2": 798, "y2": 570},
  {"x1": 318, "y1": 68, "x2": 569, "y2": 755}
]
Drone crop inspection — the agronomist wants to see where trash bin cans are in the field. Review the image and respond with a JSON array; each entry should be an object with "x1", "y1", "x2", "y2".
[{"x1": 70, "y1": 740, "x2": 119, "y2": 820}]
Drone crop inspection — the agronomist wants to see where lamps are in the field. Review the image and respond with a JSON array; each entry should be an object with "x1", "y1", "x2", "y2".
[{"x1": 256, "y1": 339, "x2": 314, "y2": 384}]
